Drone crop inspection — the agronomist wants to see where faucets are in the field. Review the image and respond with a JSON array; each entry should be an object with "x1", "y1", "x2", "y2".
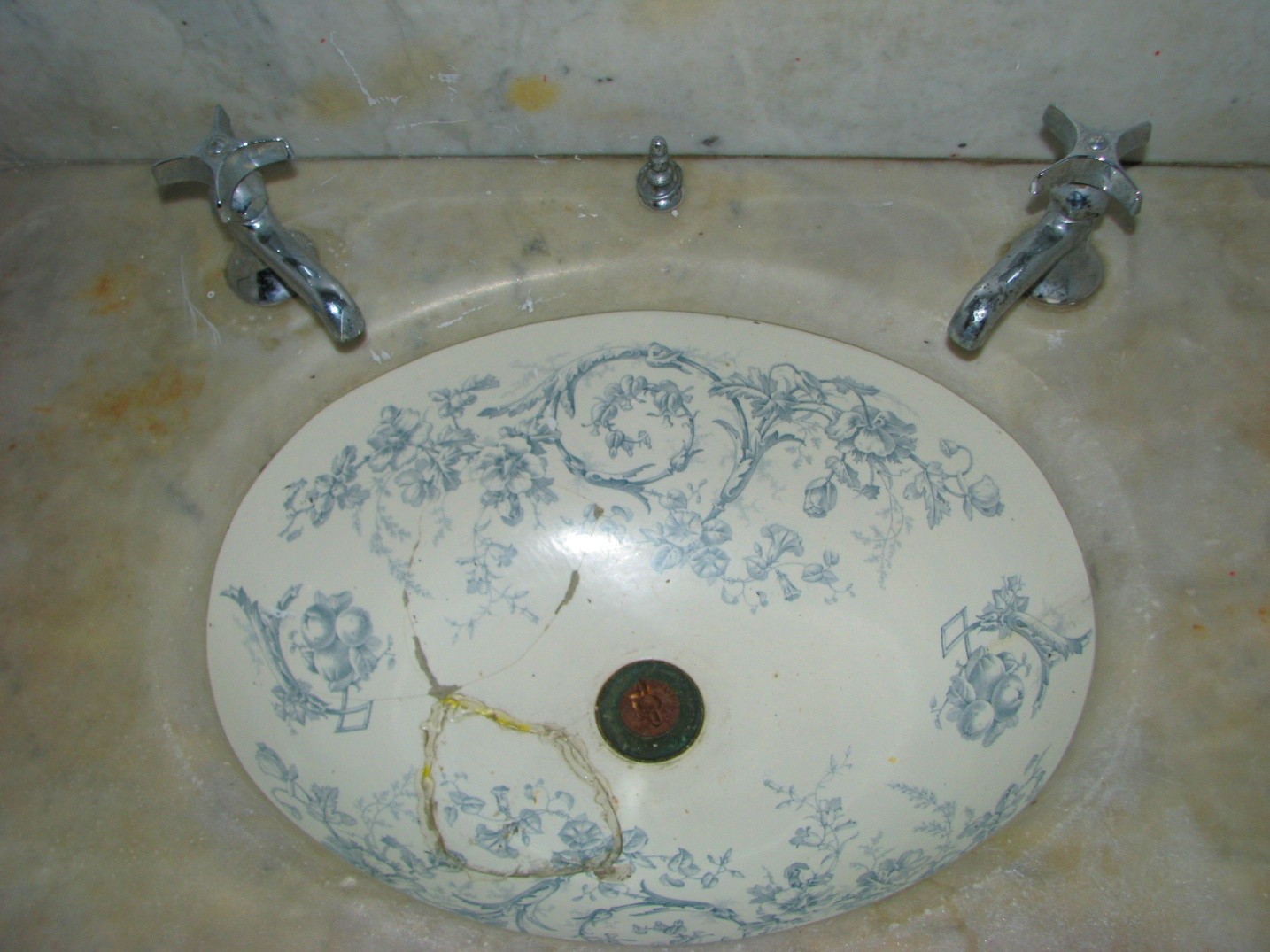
[
  {"x1": 154, "y1": 98, "x2": 372, "y2": 352},
  {"x1": 939, "y1": 105, "x2": 1162, "y2": 357}
]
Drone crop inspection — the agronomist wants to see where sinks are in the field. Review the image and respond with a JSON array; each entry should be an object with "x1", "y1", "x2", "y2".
[{"x1": 143, "y1": 253, "x2": 1153, "y2": 952}]
